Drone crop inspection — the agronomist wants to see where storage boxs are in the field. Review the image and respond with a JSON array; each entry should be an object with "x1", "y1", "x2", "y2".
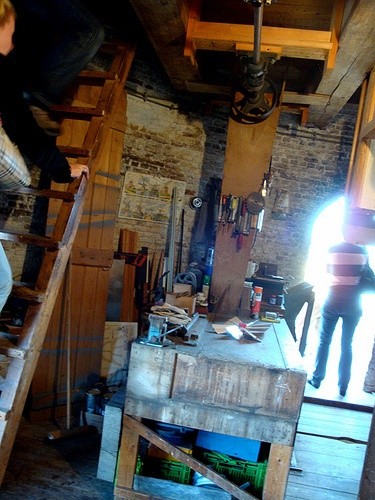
[{"x1": 134, "y1": 417, "x2": 268, "y2": 496}]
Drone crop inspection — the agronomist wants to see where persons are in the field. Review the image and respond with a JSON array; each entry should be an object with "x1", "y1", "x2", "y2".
[
  {"x1": 307, "y1": 221, "x2": 369, "y2": 396},
  {"x1": 362, "y1": 338, "x2": 375, "y2": 394},
  {"x1": 0, "y1": 0, "x2": 90, "y2": 317}
]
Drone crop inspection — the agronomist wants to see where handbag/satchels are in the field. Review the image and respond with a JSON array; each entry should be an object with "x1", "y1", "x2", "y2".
[{"x1": 360, "y1": 256, "x2": 375, "y2": 292}]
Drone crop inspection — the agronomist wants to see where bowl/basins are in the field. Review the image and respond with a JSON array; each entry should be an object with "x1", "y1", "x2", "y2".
[{"x1": 173, "y1": 283, "x2": 192, "y2": 296}]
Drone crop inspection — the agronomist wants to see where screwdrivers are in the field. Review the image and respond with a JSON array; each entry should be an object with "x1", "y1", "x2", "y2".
[
  {"x1": 238, "y1": 216, "x2": 244, "y2": 249},
  {"x1": 241, "y1": 198, "x2": 248, "y2": 228}
]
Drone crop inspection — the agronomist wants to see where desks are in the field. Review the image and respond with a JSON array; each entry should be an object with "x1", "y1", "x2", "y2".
[{"x1": 113, "y1": 314, "x2": 307, "y2": 500}]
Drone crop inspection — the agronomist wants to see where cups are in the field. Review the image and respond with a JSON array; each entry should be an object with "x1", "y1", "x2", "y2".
[{"x1": 246, "y1": 262, "x2": 259, "y2": 278}]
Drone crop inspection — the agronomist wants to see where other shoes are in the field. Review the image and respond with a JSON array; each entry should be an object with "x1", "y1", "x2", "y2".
[
  {"x1": 308, "y1": 380, "x2": 319, "y2": 389},
  {"x1": 340, "y1": 389, "x2": 347, "y2": 396}
]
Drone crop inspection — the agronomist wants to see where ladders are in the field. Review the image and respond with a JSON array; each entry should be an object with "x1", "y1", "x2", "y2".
[{"x1": 1, "y1": 27, "x2": 139, "y2": 483}]
